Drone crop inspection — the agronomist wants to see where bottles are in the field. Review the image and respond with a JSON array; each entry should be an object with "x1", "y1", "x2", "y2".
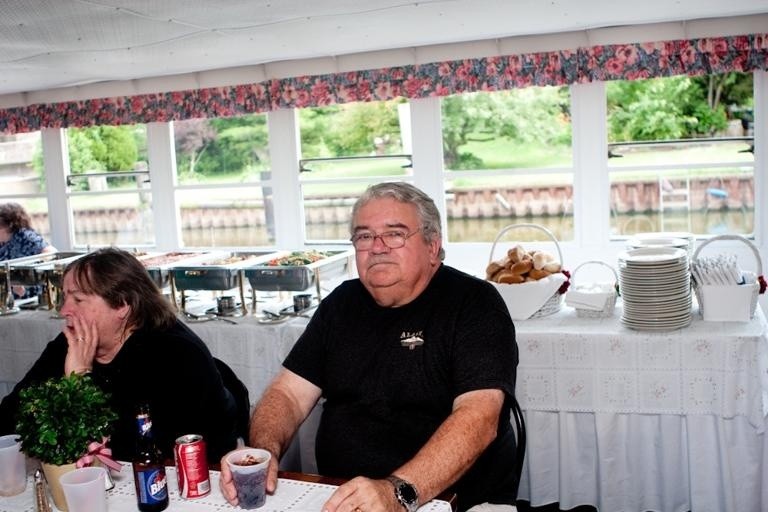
[{"x1": 131, "y1": 401, "x2": 170, "y2": 512}]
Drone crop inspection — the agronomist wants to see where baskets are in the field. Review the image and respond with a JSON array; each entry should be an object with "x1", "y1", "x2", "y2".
[
  {"x1": 486, "y1": 223, "x2": 565, "y2": 320},
  {"x1": 569, "y1": 260, "x2": 619, "y2": 318},
  {"x1": 690, "y1": 234, "x2": 762, "y2": 319}
]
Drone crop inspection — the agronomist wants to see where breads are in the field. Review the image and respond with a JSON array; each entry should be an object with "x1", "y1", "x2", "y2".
[{"x1": 486, "y1": 243, "x2": 559, "y2": 283}]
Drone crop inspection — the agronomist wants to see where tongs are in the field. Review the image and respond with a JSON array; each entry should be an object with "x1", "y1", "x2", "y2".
[{"x1": 0, "y1": 259, "x2": 15, "y2": 311}]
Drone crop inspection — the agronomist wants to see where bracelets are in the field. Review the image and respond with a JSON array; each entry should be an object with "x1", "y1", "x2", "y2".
[{"x1": 74, "y1": 368, "x2": 93, "y2": 376}]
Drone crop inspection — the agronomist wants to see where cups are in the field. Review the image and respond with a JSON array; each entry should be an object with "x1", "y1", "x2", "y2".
[
  {"x1": 57, "y1": 465, "x2": 107, "y2": 512},
  {"x1": 225, "y1": 448, "x2": 272, "y2": 509},
  {"x1": 0, "y1": 433, "x2": 29, "y2": 497}
]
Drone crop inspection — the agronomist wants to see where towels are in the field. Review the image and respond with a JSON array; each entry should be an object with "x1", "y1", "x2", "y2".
[
  {"x1": 563, "y1": 280, "x2": 612, "y2": 311},
  {"x1": 687, "y1": 255, "x2": 746, "y2": 286}
]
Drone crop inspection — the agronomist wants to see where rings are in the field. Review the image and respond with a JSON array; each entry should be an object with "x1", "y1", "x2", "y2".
[{"x1": 76, "y1": 338, "x2": 85, "y2": 341}]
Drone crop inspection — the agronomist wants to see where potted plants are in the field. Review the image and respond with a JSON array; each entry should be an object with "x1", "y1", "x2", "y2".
[{"x1": 13, "y1": 369, "x2": 125, "y2": 512}]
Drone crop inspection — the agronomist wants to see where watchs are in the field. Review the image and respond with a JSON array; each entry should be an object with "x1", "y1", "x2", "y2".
[{"x1": 383, "y1": 473, "x2": 421, "y2": 512}]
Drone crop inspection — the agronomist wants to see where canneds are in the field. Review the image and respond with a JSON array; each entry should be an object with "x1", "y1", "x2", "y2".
[{"x1": 174, "y1": 434, "x2": 211, "y2": 500}]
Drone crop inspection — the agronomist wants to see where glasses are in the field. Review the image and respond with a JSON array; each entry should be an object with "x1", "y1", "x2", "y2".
[{"x1": 350, "y1": 226, "x2": 428, "y2": 251}]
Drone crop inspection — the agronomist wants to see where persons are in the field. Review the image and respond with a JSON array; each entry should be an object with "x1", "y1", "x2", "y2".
[
  {"x1": 219, "y1": 183, "x2": 522, "y2": 512},
  {"x1": 0, "y1": 247, "x2": 241, "y2": 472},
  {"x1": 0, "y1": 201, "x2": 61, "y2": 260}
]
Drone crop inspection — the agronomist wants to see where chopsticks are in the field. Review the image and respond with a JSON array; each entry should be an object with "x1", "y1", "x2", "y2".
[{"x1": 691, "y1": 253, "x2": 746, "y2": 287}]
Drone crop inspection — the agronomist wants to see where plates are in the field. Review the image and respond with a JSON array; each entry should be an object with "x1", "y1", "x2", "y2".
[
  {"x1": 0, "y1": 306, "x2": 20, "y2": 316},
  {"x1": 617, "y1": 229, "x2": 696, "y2": 332}
]
